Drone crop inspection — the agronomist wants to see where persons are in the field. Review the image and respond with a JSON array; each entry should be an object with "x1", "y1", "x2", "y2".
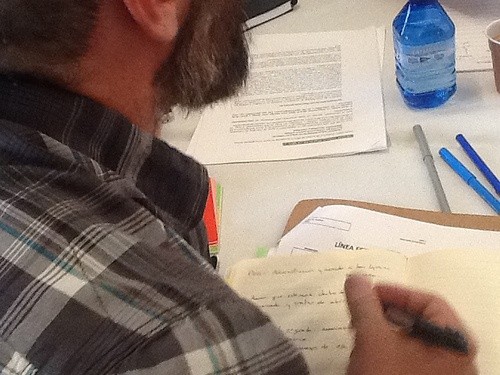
[{"x1": 0, "y1": 0, "x2": 479, "y2": 375}]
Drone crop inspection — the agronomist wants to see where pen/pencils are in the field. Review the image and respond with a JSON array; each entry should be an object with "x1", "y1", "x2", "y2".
[
  {"x1": 457, "y1": 132, "x2": 500, "y2": 196},
  {"x1": 380, "y1": 302, "x2": 474, "y2": 357},
  {"x1": 412, "y1": 124, "x2": 454, "y2": 216},
  {"x1": 437, "y1": 147, "x2": 500, "y2": 217}
]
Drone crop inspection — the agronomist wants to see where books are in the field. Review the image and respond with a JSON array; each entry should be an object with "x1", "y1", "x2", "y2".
[
  {"x1": 206, "y1": 179, "x2": 223, "y2": 254},
  {"x1": 241, "y1": 0, "x2": 297, "y2": 31}
]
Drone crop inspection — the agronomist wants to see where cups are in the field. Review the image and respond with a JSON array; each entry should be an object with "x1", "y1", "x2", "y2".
[{"x1": 486, "y1": 19, "x2": 500, "y2": 93}]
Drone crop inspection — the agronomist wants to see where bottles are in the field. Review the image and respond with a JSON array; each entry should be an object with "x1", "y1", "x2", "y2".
[{"x1": 392, "y1": 0, "x2": 457, "y2": 108}]
